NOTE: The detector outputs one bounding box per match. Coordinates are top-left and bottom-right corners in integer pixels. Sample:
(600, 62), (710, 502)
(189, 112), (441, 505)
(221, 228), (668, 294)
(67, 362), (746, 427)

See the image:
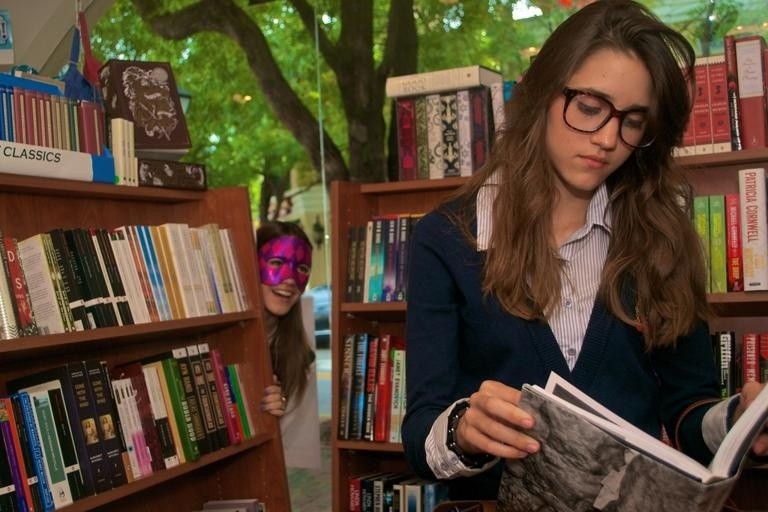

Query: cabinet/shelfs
(330, 149), (768, 511)
(0, 174), (294, 512)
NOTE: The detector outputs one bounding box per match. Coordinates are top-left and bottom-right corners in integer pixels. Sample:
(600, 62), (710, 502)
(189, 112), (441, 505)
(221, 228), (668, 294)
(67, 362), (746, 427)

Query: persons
(395, 1), (768, 511)
(259, 219), (317, 418)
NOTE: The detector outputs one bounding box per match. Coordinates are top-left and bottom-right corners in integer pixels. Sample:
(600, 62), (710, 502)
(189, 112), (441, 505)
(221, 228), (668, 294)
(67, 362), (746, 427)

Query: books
(140, 159), (208, 190)
(671, 33), (768, 158)
(1, 84), (104, 155)
(0, 342), (256, 512)
(691, 165), (768, 294)
(336, 329), (407, 444)
(105, 117), (139, 187)
(386, 63), (524, 181)
(348, 463), (448, 512)
(708, 326), (768, 400)
(495, 369), (768, 511)
(0, 220), (252, 341)
(344, 212), (427, 302)
(98, 57), (192, 163)
(201, 498), (266, 512)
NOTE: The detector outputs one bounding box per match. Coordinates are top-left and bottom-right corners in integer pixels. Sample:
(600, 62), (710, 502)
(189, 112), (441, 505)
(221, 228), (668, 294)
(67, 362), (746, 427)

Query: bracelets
(447, 403), (496, 470)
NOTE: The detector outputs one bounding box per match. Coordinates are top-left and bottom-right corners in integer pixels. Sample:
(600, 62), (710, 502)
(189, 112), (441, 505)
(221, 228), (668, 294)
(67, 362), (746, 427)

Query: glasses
(561, 86), (660, 149)
